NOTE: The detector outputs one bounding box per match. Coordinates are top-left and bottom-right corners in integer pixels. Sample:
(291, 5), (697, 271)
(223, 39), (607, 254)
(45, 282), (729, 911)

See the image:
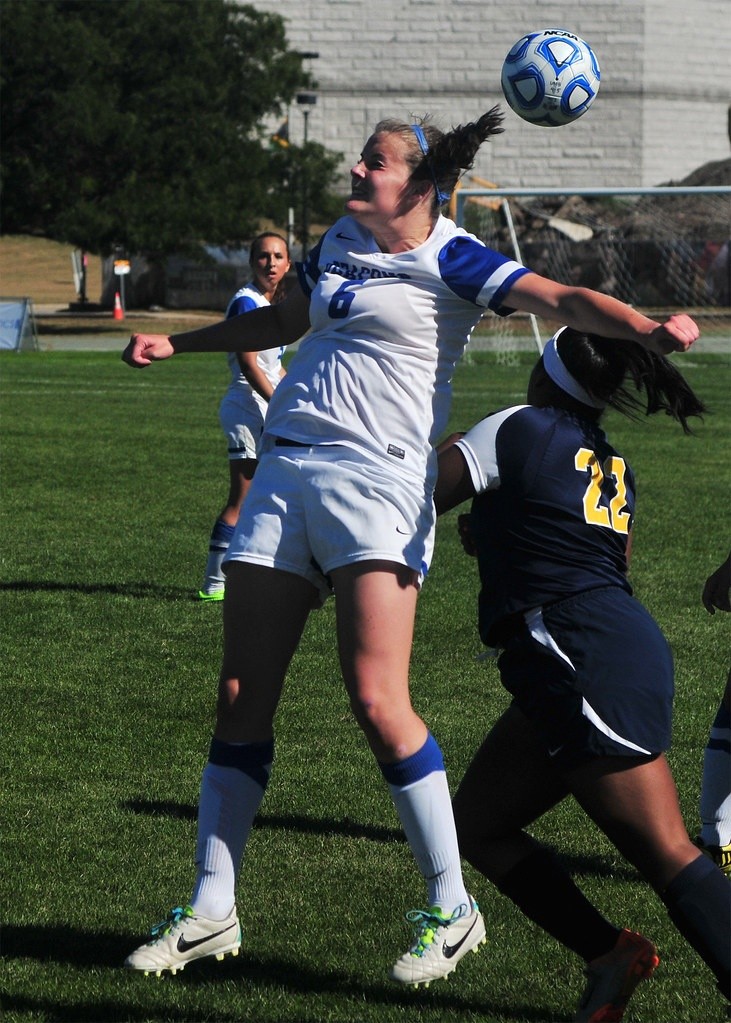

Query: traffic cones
(112, 292), (125, 319)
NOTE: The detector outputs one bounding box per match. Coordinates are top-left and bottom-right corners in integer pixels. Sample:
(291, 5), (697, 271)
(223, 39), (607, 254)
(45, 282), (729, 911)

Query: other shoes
(198, 580), (225, 601)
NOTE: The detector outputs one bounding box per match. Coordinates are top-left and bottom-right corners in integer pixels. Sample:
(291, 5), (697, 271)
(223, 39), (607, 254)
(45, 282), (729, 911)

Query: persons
(434, 324), (731, 1023)
(689, 551), (731, 871)
(198, 232), (287, 602)
(121, 104), (701, 989)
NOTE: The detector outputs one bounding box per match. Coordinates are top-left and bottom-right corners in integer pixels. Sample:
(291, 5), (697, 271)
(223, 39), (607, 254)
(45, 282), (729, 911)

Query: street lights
(297, 54), (318, 261)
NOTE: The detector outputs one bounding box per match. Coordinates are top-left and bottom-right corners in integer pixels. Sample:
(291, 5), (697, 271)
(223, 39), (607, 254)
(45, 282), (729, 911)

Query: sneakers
(573, 928), (659, 1023)
(124, 903), (242, 979)
(391, 894), (486, 991)
(691, 835), (731, 880)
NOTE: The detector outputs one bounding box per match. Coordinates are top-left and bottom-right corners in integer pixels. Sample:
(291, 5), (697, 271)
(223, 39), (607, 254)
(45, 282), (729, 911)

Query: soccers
(499, 29), (604, 129)
